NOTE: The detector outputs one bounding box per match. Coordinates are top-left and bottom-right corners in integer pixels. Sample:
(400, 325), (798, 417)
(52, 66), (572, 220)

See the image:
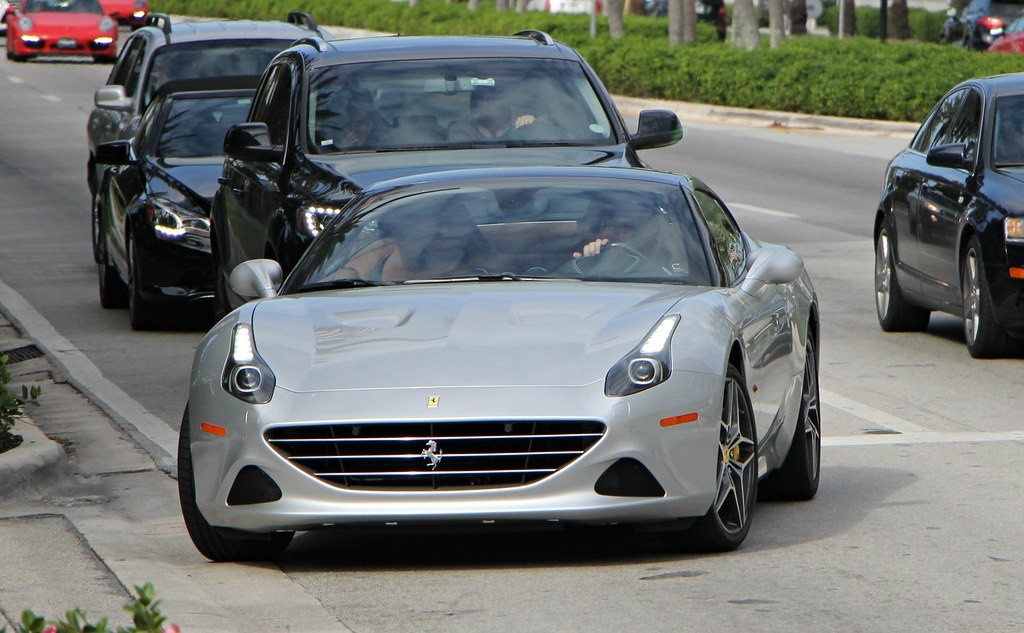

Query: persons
(553, 195), (671, 276)
(448, 87), (540, 144)
(337, 107), (383, 148)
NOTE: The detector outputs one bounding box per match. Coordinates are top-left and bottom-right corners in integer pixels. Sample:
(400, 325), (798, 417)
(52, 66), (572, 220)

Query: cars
(177, 165), (822, 562)
(873, 72), (1024, 359)
(0, 0), (148, 63)
(941, 0), (1024, 53)
(642, 0), (727, 41)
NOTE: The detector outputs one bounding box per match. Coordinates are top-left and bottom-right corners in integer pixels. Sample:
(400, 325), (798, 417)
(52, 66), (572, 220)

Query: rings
(524, 119), (529, 123)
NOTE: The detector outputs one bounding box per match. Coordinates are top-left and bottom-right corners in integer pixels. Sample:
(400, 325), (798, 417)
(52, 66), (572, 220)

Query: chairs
(383, 201), (503, 275)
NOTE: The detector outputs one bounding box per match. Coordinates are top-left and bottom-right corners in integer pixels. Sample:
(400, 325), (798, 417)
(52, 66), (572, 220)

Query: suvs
(87, 13), (683, 330)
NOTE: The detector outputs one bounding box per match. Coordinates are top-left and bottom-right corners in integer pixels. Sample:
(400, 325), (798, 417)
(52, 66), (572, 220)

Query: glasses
(575, 217), (614, 235)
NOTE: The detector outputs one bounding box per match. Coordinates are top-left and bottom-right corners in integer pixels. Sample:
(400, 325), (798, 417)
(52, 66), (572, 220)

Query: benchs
(372, 87), (466, 125)
(481, 222), (581, 270)
